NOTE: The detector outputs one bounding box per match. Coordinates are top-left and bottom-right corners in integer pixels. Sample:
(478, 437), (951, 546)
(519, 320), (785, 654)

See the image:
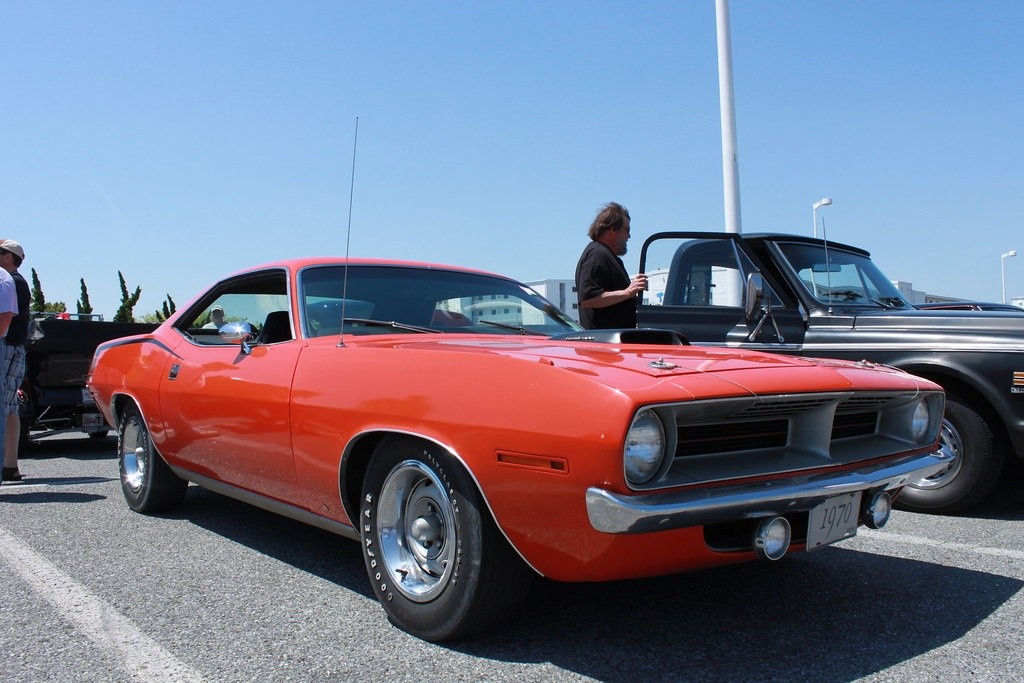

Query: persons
(202, 305), (228, 329)
(574, 202), (648, 330)
(0, 238), (31, 482)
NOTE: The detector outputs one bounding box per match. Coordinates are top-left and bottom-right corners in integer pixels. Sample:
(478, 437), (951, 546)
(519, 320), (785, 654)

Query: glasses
(621, 226), (630, 234)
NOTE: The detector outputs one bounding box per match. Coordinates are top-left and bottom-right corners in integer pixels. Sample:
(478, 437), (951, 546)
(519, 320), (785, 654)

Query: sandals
(1, 466), (22, 481)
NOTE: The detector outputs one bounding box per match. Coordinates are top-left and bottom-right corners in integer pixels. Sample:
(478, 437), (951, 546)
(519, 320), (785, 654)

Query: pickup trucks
(515, 226), (1024, 516)
(17, 310), (222, 459)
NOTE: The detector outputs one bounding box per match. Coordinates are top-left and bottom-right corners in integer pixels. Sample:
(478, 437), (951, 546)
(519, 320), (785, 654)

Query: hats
(0, 239), (25, 260)
(211, 305), (224, 313)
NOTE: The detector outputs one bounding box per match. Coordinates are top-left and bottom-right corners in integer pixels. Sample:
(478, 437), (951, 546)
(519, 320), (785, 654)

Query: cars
(83, 256), (959, 647)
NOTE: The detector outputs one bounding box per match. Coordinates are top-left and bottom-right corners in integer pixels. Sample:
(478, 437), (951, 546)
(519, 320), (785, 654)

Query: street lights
(1001, 251), (1017, 304)
(813, 197), (833, 238)
